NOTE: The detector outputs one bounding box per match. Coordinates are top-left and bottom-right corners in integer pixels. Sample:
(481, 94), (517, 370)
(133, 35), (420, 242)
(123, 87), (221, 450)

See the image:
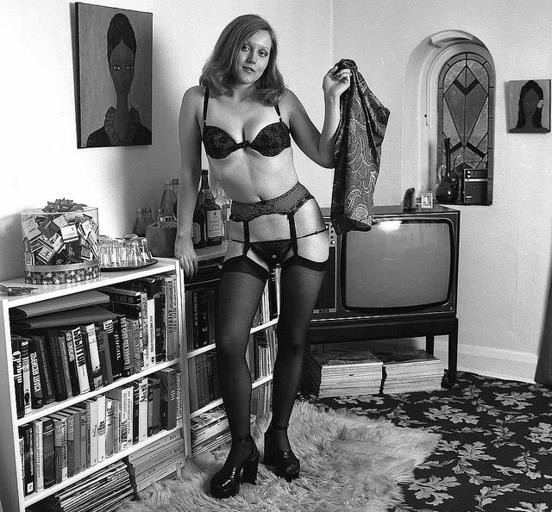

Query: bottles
(172, 176), (180, 202)
(200, 187), (225, 247)
(194, 169), (217, 209)
(189, 208), (209, 250)
(436, 138), (459, 203)
(161, 178), (178, 224)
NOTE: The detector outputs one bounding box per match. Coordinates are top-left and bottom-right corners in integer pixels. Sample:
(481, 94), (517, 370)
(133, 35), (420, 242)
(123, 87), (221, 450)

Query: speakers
(313, 206), (338, 320)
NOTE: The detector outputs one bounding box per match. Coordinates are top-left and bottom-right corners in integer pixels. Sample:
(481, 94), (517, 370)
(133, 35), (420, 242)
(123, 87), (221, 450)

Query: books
(189, 352), (229, 405)
(304, 343), (446, 399)
(6, 276), (194, 420)
(2, 363), (180, 507)
(245, 332), (297, 379)
(192, 385), (282, 456)
(183, 277), (217, 335)
(250, 278), (269, 322)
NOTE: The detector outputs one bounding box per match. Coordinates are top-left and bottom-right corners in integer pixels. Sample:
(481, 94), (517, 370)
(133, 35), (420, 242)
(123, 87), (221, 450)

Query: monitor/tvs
(339, 204), (461, 318)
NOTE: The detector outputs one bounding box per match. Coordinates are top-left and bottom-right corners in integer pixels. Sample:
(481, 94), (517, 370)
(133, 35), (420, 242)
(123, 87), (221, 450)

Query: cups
(99, 233), (152, 268)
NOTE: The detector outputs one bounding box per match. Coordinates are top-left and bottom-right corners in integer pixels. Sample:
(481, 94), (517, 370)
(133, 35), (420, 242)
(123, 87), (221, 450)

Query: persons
(505, 82), (550, 133)
(77, 2), (151, 141)
(174, 14), (353, 497)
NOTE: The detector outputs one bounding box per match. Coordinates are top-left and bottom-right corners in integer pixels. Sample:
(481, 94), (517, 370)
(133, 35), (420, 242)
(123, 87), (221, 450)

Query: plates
(100, 259), (158, 272)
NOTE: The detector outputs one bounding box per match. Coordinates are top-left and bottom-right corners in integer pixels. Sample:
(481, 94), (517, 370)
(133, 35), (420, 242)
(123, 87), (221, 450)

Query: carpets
(122, 401), (441, 511)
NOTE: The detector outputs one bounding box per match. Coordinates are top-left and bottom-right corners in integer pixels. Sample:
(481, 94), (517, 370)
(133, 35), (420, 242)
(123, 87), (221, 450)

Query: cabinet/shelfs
(156, 240), (281, 457)
(304, 318), (458, 398)
(1, 255), (188, 509)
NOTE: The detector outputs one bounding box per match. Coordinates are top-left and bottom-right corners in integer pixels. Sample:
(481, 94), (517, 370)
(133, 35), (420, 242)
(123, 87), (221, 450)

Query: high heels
(263, 426), (300, 481)
(210, 432), (260, 499)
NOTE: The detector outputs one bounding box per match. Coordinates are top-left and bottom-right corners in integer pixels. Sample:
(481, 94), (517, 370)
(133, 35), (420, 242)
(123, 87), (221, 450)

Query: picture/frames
(420, 193), (433, 208)
